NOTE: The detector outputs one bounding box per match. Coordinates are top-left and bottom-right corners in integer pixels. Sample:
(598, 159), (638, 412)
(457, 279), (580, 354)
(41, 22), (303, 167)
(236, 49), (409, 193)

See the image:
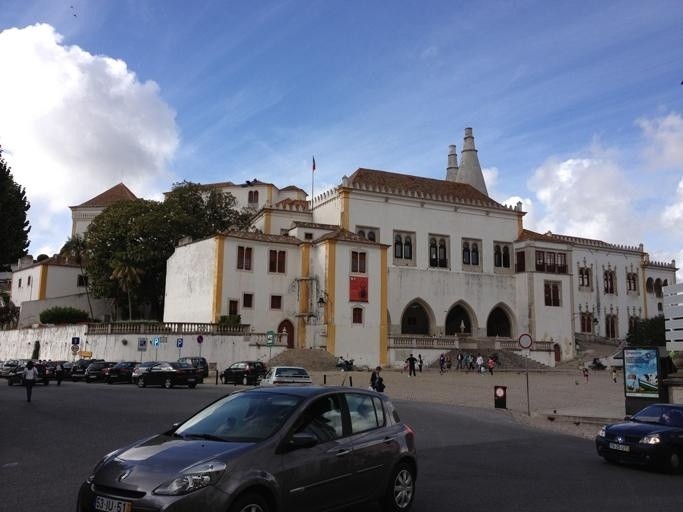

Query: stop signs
(518, 334), (532, 348)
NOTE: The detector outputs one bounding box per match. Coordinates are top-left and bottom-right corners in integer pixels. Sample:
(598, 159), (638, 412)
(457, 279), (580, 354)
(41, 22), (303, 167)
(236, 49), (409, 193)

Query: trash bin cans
(495, 386), (507, 409)
(208, 363), (216, 376)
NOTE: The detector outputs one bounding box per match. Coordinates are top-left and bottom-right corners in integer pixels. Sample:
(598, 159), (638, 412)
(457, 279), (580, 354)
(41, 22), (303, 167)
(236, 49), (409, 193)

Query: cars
(75, 385), (418, 511)
(258, 366), (312, 387)
(595, 403), (682, 474)
(0, 357), (208, 389)
(220, 361), (267, 386)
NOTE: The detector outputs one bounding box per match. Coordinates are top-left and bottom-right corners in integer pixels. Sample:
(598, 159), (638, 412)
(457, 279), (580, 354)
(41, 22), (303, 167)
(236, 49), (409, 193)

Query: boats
(638, 378), (658, 391)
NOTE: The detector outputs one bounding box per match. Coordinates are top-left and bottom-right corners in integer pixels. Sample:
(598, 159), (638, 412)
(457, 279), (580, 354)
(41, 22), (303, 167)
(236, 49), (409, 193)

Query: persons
(39, 359), (63, 385)
(404, 361), (408, 372)
(24, 361), (38, 402)
(439, 351), (495, 375)
(338, 356), (347, 371)
(613, 370), (617, 383)
(583, 368), (589, 383)
(417, 354), (423, 372)
(371, 367), (381, 391)
(376, 377), (385, 392)
(407, 354), (417, 377)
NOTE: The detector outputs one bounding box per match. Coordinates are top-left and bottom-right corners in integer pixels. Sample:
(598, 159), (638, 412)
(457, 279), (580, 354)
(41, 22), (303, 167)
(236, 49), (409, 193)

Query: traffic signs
(267, 330), (273, 347)
(77, 351), (92, 357)
(154, 338), (160, 347)
(177, 338), (183, 348)
